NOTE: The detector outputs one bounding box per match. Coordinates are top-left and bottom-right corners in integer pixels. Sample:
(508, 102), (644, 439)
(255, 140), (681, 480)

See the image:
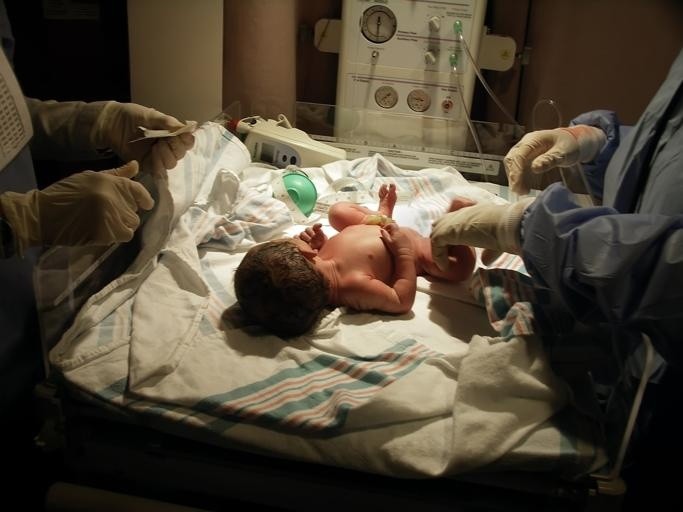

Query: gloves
(430, 196), (538, 273)
(503, 124), (607, 196)
(1, 159), (155, 258)
(25, 96), (195, 169)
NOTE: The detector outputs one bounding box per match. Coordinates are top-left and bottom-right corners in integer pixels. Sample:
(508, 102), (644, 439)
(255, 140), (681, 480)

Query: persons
(234, 184), (477, 338)
(429, 47), (683, 512)
(0, 1), (196, 512)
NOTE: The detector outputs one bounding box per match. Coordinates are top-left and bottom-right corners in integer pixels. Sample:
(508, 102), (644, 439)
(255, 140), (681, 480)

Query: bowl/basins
(280, 173), (317, 217)
